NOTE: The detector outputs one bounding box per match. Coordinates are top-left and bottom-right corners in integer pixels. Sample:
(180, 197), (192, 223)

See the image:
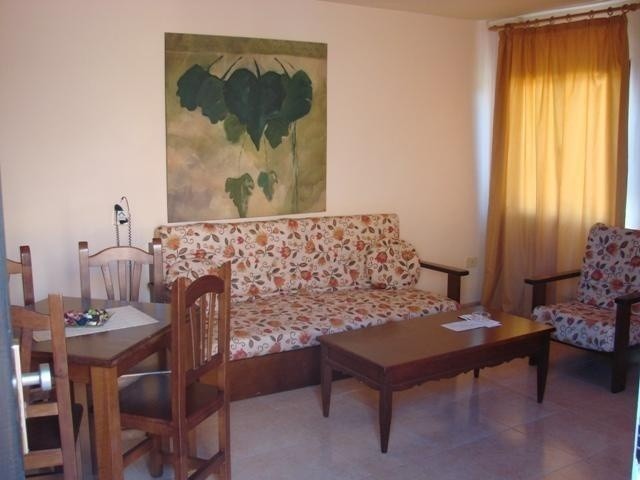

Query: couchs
(151, 214), (468, 402)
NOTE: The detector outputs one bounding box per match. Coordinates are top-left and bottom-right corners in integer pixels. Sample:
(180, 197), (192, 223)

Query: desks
(8, 291), (204, 476)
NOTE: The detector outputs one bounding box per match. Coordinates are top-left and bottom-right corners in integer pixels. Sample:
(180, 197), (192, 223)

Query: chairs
(5, 241), (38, 313)
(72, 238), (168, 304)
(8, 292), (89, 480)
(106, 259), (233, 475)
(525, 223), (640, 393)
